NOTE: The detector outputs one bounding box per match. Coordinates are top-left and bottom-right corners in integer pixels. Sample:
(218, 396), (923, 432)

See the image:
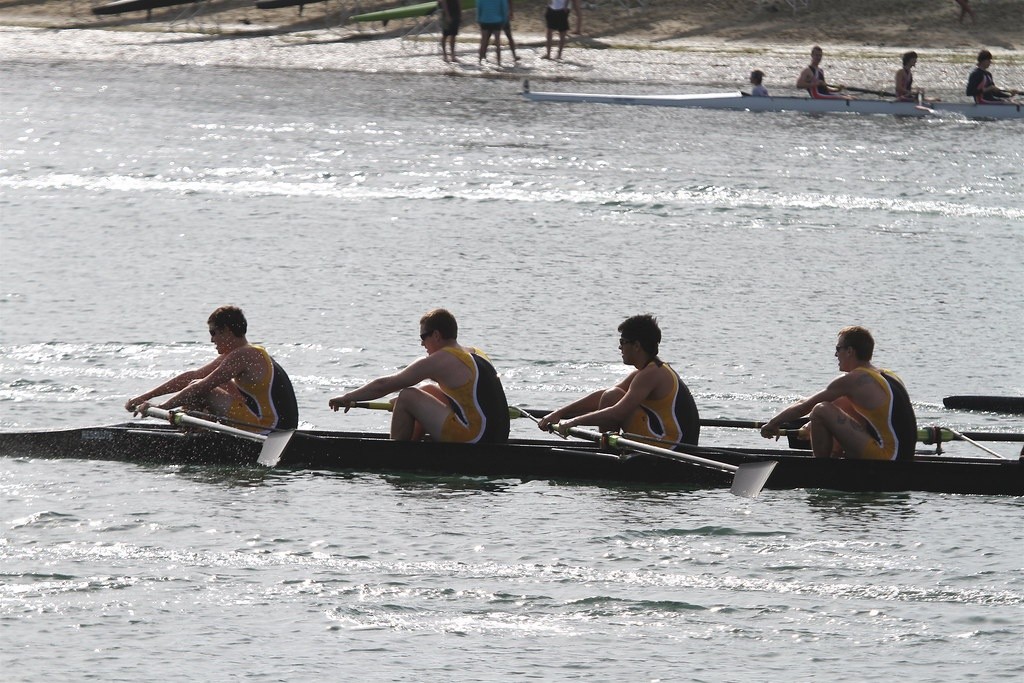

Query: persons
(966, 50), (1014, 104)
(896, 51), (924, 101)
(760, 326), (917, 460)
(749, 70), (768, 95)
(126, 306), (298, 434)
(955, 0), (976, 27)
(328, 308), (510, 442)
(797, 45), (859, 98)
(438, 0), (582, 66)
(537, 315), (700, 452)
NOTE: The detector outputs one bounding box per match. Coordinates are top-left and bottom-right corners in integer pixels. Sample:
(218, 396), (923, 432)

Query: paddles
(538, 421), (780, 499)
(825, 83), (896, 97)
(998, 88), (1024, 96)
(128, 403), (298, 469)
(335, 401), (813, 449)
(762, 426), (1024, 446)
(916, 92), (930, 111)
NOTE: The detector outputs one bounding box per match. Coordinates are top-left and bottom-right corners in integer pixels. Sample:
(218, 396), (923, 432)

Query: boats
(942, 394), (1024, 415)
(90, 0), (330, 16)
(349, 0), (477, 22)
(518, 78), (1024, 124)
(0, 418), (1024, 500)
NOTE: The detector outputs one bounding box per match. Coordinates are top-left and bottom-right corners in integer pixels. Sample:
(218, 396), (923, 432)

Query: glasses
(619, 338), (631, 345)
(835, 345), (845, 352)
(209, 328), (219, 337)
(420, 333), (428, 340)
